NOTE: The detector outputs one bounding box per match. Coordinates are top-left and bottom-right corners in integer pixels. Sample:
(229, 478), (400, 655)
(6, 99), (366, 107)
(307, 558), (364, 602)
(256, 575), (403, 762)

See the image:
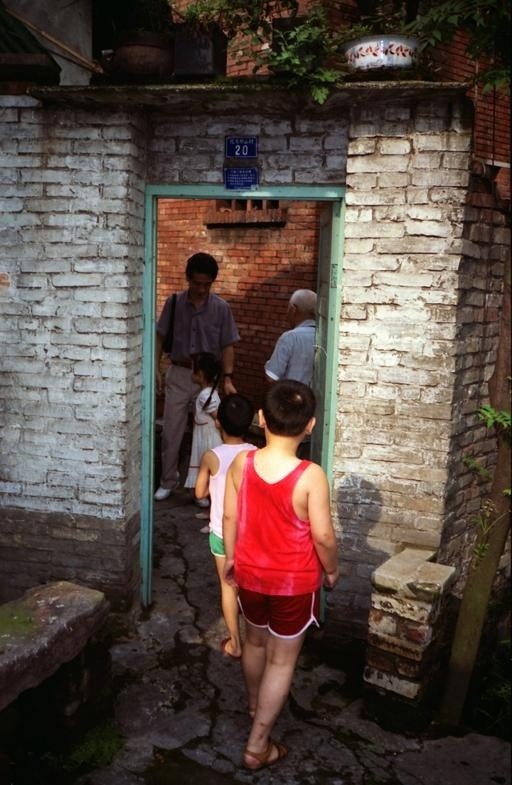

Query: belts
(172, 359), (192, 369)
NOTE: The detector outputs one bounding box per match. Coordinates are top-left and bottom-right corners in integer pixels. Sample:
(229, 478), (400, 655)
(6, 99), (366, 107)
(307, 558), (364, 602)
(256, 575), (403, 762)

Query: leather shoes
(200, 526), (208, 533)
(195, 512), (209, 519)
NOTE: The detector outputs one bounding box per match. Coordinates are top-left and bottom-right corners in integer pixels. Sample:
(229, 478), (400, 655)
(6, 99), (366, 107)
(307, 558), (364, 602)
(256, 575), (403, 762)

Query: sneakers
(154, 479), (179, 503)
(181, 488), (210, 508)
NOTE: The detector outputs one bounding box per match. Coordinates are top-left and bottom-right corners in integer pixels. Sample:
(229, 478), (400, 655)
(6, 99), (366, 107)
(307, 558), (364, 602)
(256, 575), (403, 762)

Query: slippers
(221, 637), (242, 659)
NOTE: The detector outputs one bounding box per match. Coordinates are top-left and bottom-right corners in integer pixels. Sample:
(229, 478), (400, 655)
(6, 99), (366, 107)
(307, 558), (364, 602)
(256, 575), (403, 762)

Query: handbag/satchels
(162, 293), (177, 355)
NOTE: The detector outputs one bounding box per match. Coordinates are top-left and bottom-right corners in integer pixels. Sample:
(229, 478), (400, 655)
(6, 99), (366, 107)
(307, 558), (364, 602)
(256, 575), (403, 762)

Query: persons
(184, 353), (222, 535)
(263, 289), (320, 463)
(194, 392), (259, 659)
(221, 377), (342, 772)
(154, 251), (241, 502)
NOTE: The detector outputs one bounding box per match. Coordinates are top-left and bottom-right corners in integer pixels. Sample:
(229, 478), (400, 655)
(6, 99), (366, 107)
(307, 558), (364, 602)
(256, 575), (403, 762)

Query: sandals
(245, 701), (288, 773)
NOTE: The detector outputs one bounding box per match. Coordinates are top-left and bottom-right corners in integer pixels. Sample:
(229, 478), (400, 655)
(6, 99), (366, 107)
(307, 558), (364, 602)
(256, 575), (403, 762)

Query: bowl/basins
(336, 32), (423, 78)
(112, 43), (175, 82)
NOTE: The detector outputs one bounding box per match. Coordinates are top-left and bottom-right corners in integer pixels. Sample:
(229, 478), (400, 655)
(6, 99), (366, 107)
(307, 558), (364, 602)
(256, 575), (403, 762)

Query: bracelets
(223, 372), (234, 379)
